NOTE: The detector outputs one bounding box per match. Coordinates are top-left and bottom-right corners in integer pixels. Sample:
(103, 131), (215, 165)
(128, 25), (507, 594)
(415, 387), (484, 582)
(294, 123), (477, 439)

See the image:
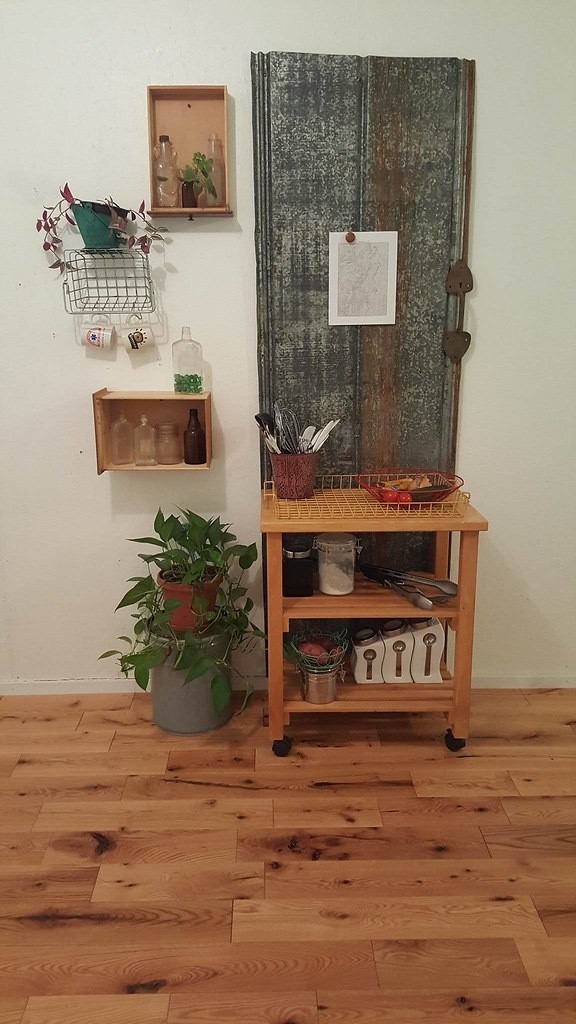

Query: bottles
(183, 409), (207, 465)
(111, 409), (134, 465)
(155, 423), (182, 464)
(204, 130), (225, 207)
(153, 135), (179, 208)
(133, 414), (157, 466)
(172, 326), (203, 395)
(349, 617), (447, 684)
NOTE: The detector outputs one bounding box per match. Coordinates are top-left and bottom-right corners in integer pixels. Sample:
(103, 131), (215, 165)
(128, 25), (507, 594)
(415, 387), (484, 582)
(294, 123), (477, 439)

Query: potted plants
(96, 504), (269, 717)
(36, 183), (170, 278)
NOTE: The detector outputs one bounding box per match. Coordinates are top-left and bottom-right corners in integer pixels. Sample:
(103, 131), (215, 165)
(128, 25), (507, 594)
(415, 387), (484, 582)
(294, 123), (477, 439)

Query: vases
(270, 453), (318, 499)
(181, 152), (217, 209)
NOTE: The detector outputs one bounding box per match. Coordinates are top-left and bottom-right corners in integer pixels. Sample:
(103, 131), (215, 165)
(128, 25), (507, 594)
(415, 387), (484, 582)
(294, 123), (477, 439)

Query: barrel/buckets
(146, 607), (234, 735)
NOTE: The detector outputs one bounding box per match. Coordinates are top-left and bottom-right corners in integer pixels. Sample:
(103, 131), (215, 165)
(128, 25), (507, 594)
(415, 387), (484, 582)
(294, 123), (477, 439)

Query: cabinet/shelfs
(92, 387), (213, 476)
(259, 489), (488, 758)
(146, 84), (234, 221)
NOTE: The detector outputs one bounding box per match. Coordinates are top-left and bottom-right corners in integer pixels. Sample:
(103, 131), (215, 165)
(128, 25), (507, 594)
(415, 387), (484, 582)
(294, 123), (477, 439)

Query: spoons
(397, 580), (450, 601)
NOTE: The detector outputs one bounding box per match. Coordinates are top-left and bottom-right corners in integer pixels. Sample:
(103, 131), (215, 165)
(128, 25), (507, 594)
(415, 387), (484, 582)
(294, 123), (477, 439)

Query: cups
(119, 314), (154, 353)
(313, 532), (362, 595)
(295, 663), (347, 704)
(80, 314), (115, 352)
(283, 543), (314, 597)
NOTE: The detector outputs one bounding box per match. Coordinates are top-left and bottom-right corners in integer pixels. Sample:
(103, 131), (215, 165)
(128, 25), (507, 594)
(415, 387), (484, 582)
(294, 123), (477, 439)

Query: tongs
(362, 563), (459, 610)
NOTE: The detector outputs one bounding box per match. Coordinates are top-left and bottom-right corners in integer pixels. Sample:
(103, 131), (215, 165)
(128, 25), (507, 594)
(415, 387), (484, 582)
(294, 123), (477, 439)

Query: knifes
(301, 418), (341, 453)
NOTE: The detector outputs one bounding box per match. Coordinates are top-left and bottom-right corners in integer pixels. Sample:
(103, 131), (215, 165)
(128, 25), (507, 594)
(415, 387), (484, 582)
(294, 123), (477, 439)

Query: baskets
(263, 473), (471, 519)
(291, 628), (350, 668)
(62, 247), (156, 321)
(356, 467), (464, 509)
